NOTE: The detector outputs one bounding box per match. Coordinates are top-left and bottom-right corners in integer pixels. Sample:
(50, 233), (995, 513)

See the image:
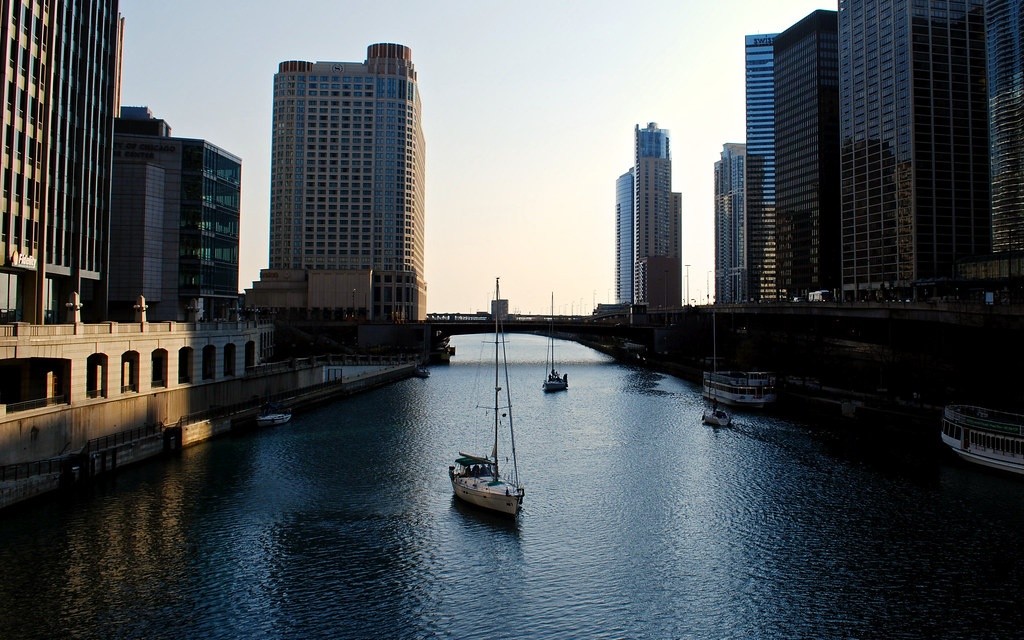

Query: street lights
(685, 264), (692, 306)
(351, 289), (360, 320)
(665, 269), (669, 322)
(707, 270), (712, 305)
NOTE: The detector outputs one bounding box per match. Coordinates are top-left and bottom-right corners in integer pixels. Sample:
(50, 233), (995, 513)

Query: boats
(702, 368), (778, 408)
(257, 404), (292, 425)
(415, 364), (431, 378)
(939, 405), (1024, 476)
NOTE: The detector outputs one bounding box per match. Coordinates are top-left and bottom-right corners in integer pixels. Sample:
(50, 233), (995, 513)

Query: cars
(791, 296), (806, 304)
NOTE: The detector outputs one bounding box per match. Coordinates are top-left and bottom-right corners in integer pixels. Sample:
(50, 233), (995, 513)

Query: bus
(809, 290), (831, 303)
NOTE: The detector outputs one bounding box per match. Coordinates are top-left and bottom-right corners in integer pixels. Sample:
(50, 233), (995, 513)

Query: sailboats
(698, 297), (730, 427)
(543, 293), (568, 391)
(449, 275), (526, 519)
